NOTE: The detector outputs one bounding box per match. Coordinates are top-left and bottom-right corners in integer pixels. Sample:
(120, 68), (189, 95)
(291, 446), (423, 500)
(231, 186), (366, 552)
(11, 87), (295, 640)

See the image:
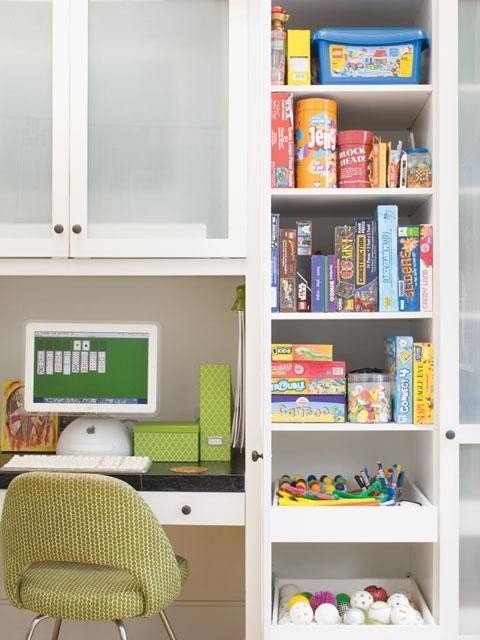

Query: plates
(0, 0), (271, 276)
(246, 0), (480, 640)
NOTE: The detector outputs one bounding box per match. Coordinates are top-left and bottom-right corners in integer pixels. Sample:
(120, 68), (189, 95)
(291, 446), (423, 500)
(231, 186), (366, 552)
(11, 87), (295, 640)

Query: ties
(23, 321), (166, 455)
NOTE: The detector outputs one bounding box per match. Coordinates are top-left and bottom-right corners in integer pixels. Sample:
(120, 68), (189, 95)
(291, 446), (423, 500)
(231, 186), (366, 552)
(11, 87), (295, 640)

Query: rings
(354, 458), (404, 500)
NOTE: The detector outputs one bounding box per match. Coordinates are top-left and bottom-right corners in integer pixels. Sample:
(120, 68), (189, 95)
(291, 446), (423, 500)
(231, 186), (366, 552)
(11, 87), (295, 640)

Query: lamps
(0, 473), (189, 640)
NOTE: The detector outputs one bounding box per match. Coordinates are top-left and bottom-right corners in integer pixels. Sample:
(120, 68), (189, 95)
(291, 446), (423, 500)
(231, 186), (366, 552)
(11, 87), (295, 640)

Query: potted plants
(1, 378), (61, 453)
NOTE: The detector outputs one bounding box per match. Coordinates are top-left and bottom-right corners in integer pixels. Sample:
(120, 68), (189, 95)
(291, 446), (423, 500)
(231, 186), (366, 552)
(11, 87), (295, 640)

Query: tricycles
(73, 340), (91, 351)
(37, 351), (106, 374)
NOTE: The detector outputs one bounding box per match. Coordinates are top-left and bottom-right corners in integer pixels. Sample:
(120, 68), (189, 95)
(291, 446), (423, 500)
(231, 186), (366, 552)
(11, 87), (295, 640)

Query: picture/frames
(1, 441), (248, 530)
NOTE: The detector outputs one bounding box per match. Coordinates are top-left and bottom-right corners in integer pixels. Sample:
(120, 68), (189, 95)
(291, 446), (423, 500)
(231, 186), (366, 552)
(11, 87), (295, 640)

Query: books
(310, 24), (432, 86)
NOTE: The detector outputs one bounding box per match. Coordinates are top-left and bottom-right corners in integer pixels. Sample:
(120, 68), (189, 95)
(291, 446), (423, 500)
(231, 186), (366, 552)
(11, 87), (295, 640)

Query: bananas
(1, 453), (153, 475)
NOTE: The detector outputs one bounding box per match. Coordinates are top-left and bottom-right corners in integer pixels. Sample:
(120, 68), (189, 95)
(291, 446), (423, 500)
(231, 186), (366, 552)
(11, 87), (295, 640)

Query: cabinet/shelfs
(295, 96), (338, 189)
(336, 128), (377, 189)
(347, 373), (393, 423)
(406, 147), (432, 189)
